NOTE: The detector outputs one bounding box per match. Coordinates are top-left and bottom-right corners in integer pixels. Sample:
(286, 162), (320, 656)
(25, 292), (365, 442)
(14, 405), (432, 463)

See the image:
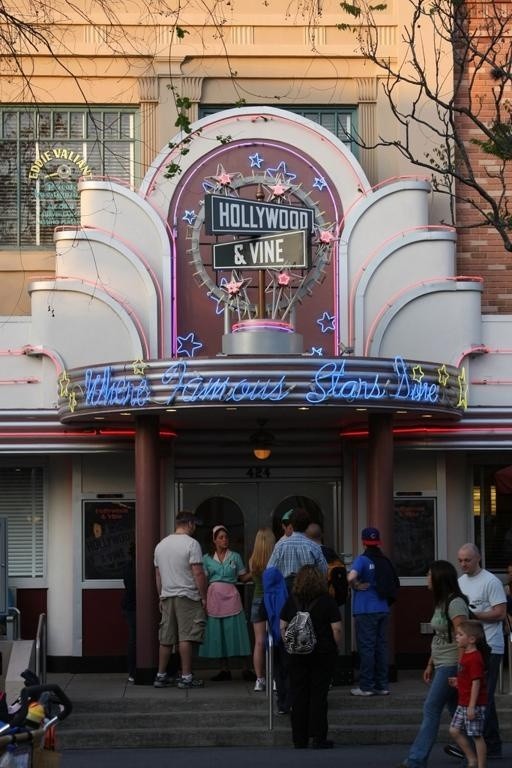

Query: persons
(400, 543), (507, 768)
(350, 527), (390, 695)
(277, 564), (345, 748)
(152, 510), (293, 691)
(306, 522), (348, 606)
(266, 508), (328, 714)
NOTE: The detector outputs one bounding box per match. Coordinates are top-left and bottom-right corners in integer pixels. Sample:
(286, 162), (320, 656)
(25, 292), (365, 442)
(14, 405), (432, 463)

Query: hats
(360, 526), (384, 547)
(211, 524), (229, 543)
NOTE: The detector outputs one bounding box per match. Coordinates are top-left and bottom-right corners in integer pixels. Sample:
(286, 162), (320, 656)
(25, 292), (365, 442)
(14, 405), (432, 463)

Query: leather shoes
(312, 739), (335, 750)
(209, 669), (233, 682)
(240, 669), (257, 682)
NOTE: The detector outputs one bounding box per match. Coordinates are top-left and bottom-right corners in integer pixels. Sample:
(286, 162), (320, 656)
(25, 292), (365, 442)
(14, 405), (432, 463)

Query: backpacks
(444, 591), (492, 672)
(362, 550), (402, 602)
(282, 593), (322, 656)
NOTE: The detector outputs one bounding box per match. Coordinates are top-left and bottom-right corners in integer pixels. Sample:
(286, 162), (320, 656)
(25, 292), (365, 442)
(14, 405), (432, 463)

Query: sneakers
(272, 678), (277, 691)
(177, 672), (205, 690)
(370, 684), (390, 696)
(486, 746), (504, 760)
(443, 741), (466, 759)
(153, 672), (182, 689)
(350, 687), (374, 697)
(253, 677), (266, 692)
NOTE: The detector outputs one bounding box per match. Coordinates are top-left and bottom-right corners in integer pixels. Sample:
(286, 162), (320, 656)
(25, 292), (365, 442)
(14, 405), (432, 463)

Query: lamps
(251, 425), (275, 461)
